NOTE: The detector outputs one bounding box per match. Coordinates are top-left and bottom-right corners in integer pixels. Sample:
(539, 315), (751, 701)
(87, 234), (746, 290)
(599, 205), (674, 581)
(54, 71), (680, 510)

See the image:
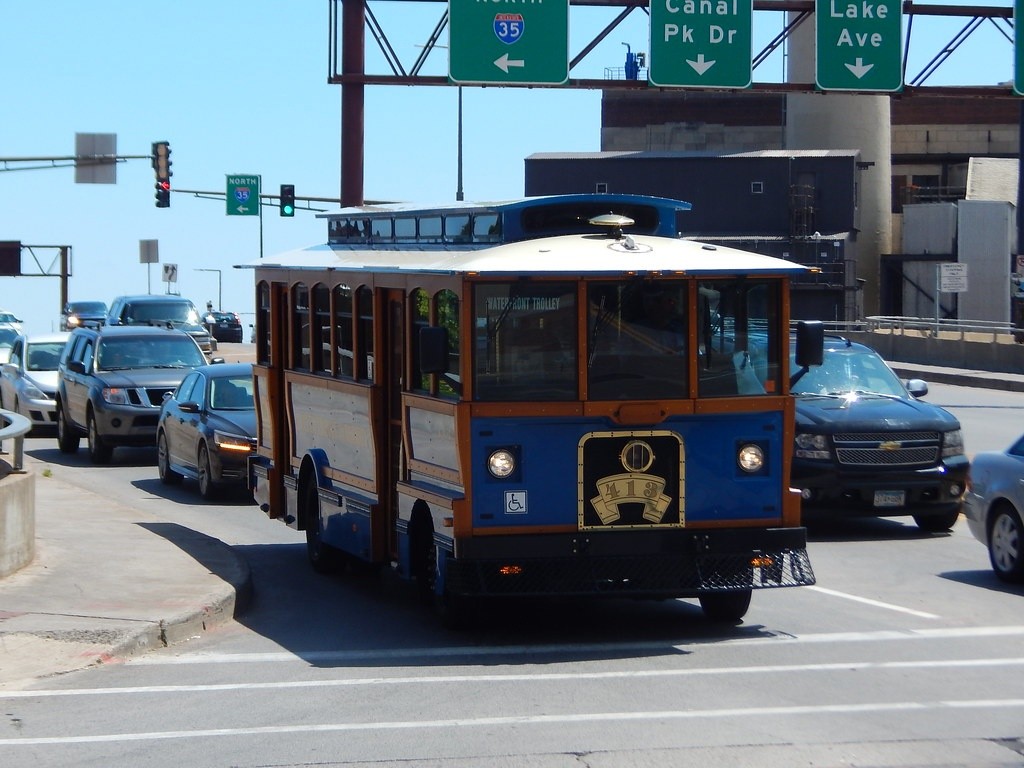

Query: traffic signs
(226, 174), (259, 216)
(448, 1), (569, 85)
(648, 1), (752, 90)
(815, 1), (903, 92)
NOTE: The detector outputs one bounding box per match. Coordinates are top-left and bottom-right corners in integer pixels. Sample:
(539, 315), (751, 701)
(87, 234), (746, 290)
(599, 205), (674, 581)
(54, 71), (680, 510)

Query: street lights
(195, 268), (222, 311)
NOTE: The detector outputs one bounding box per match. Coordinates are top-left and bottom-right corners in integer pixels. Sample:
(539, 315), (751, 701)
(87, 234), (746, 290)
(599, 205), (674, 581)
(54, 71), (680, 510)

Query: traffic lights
(280, 184), (295, 217)
(154, 141), (170, 207)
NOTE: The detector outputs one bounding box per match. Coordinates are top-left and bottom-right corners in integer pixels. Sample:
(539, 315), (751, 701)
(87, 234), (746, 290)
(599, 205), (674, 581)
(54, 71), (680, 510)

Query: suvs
(103, 294), (213, 357)
(713, 329), (970, 532)
(55, 319), (225, 465)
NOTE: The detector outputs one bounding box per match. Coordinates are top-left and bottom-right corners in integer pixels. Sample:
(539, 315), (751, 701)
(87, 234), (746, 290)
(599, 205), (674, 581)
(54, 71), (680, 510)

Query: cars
(249, 323), (256, 343)
(0, 311), (24, 364)
(156, 360), (257, 499)
(202, 311), (242, 343)
(0, 332), (73, 437)
(964, 432), (1024, 586)
(59, 301), (109, 328)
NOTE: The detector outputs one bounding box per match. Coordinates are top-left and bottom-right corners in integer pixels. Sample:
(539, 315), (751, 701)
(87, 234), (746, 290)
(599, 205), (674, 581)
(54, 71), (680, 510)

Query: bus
(232, 194), (816, 621)
